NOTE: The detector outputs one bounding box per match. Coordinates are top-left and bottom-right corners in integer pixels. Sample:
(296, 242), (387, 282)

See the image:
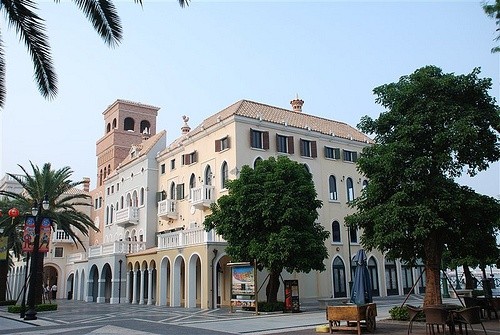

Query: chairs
(450, 306), (487, 335)
(425, 306), (454, 335)
(405, 304), (427, 335)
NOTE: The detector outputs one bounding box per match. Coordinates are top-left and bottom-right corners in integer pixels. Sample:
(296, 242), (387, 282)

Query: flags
(0, 235), (9, 261)
(39, 217), (51, 253)
(22, 217), (35, 252)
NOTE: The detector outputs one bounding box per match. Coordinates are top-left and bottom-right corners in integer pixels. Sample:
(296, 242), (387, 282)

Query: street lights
(22, 190), (49, 319)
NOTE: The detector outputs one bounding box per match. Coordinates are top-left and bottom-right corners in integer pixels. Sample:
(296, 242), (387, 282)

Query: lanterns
(8, 208), (20, 220)
(0, 211), (3, 218)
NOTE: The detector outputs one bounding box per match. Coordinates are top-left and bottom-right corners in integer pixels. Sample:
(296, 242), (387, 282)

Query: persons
(42, 282), (45, 288)
(43, 284), (49, 300)
(50, 284), (57, 300)
